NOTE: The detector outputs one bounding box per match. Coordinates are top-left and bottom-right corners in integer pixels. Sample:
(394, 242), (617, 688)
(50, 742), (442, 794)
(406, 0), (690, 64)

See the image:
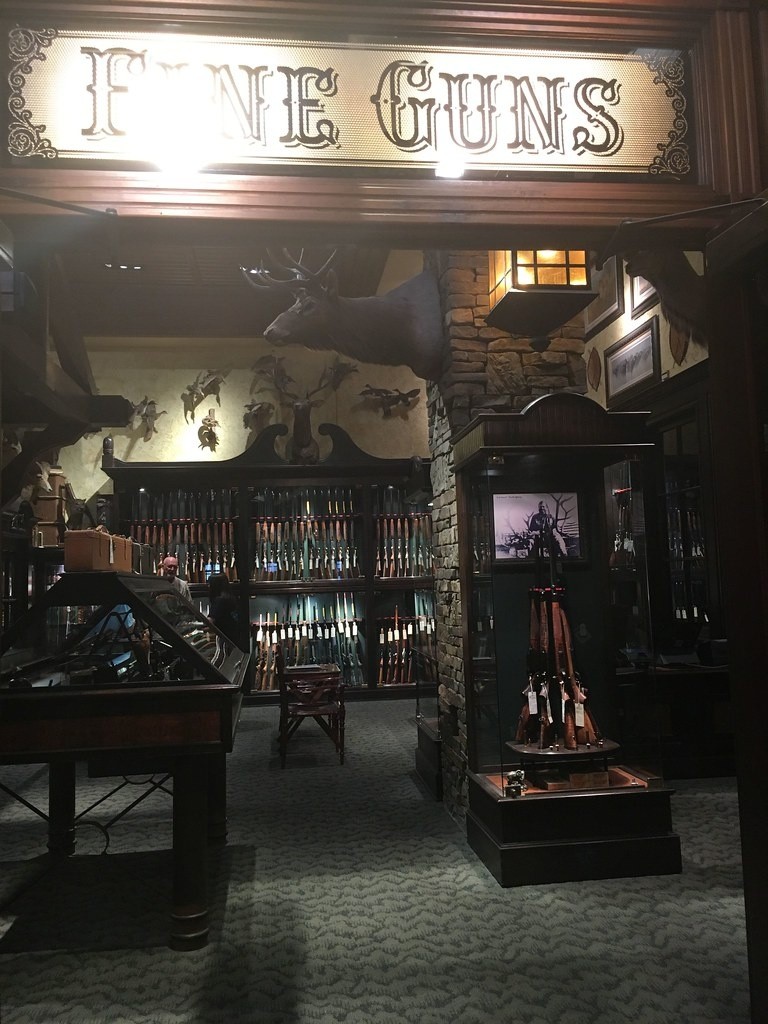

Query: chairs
(276, 641), (347, 769)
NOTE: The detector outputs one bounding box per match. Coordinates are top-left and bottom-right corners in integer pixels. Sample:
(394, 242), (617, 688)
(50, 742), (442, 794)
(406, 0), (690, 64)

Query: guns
(413, 509), (437, 680)
(126, 485), (411, 692)
(471, 507), (494, 658)
(514, 588), (604, 750)
(601, 461), (711, 640)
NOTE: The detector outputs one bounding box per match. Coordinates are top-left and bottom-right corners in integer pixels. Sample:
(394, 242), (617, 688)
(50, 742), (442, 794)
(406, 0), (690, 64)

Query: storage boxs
(63, 530), (132, 572)
(133, 543), (158, 575)
(29, 547), (64, 565)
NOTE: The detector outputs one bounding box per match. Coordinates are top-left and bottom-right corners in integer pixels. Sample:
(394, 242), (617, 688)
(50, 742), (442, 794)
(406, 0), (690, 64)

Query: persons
(65, 624), (88, 649)
(190, 572), (240, 656)
(527, 500), (555, 549)
(152, 556), (193, 606)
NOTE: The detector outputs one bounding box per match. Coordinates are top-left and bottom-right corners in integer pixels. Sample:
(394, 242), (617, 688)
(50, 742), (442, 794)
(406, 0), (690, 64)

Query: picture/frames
(584, 255), (625, 343)
(629, 275), (660, 320)
(603, 313), (662, 409)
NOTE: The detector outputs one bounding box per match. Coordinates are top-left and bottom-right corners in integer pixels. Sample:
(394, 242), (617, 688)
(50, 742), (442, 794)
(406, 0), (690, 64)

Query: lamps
(483, 250), (600, 354)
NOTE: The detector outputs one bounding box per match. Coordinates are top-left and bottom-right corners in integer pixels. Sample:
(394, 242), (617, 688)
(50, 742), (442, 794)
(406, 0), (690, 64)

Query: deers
(237, 245), (446, 384)
